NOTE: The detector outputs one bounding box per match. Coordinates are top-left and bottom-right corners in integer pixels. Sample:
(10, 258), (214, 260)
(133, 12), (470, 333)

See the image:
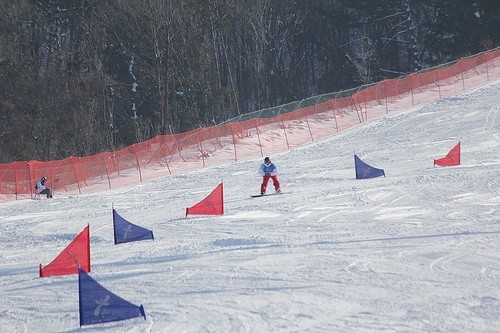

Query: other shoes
(277, 189), (282, 193)
(261, 191), (266, 195)
(46, 194), (53, 198)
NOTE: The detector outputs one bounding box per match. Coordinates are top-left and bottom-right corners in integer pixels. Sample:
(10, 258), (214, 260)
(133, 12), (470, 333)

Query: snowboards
(250, 191), (289, 198)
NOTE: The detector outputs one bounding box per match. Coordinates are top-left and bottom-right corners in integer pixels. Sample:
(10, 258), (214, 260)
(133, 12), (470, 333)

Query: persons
(34, 176), (53, 199)
(258, 157), (282, 196)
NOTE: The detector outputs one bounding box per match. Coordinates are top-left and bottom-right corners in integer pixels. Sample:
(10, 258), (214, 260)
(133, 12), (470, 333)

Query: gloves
(266, 172), (271, 176)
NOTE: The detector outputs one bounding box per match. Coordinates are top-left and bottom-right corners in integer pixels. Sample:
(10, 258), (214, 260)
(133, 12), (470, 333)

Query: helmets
(265, 157), (269, 162)
(42, 176), (47, 181)
